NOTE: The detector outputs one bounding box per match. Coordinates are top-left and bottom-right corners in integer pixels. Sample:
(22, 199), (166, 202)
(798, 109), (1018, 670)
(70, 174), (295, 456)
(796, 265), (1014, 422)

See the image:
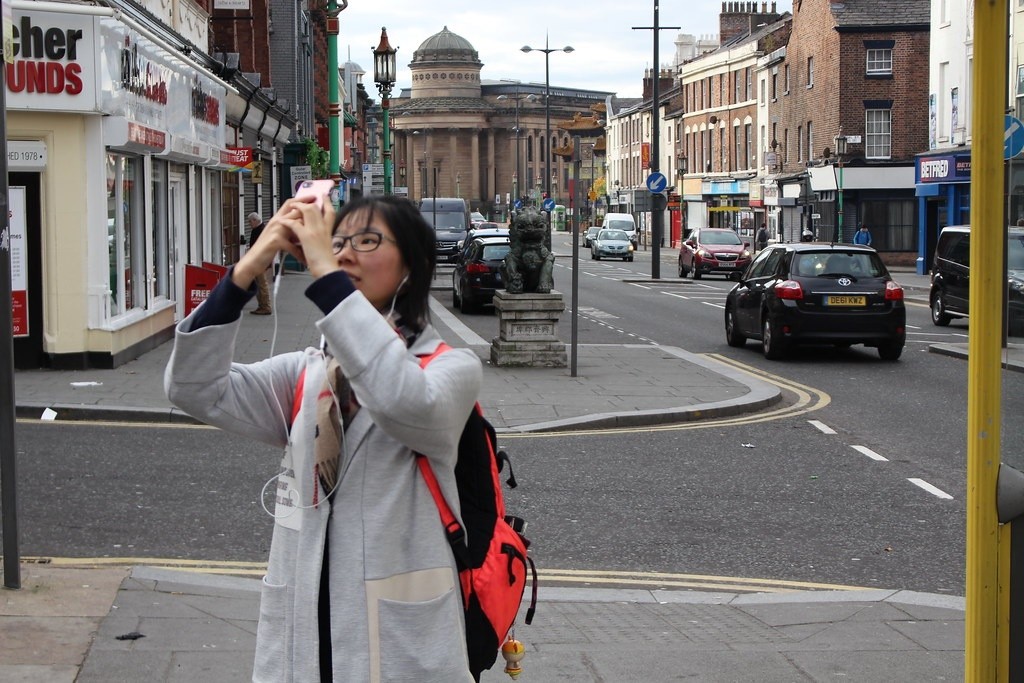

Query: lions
(497, 205), (556, 294)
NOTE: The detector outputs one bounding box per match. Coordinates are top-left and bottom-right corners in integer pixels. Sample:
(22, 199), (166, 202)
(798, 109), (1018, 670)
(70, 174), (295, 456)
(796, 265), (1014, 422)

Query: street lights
(833, 126), (848, 242)
(536, 174), (544, 201)
(520, 27), (575, 250)
(370, 26), (400, 196)
(512, 171), (518, 203)
(496, 80), (536, 215)
(456, 170), (462, 197)
(552, 172), (559, 204)
(677, 148), (687, 246)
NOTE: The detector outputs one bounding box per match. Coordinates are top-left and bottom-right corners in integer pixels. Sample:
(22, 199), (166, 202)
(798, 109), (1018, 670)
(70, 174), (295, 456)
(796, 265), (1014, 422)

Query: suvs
(677, 226), (752, 279)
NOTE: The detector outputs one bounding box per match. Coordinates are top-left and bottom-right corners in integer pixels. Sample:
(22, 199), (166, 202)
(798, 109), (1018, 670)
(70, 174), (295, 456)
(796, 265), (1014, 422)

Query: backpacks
(292, 343), (537, 683)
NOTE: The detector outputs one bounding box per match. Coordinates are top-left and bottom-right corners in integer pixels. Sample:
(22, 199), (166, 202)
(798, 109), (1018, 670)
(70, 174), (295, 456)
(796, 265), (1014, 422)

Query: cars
(447, 236), (512, 314)
(582, 226), (603, 248)
(590, 228), (634, 263)
(724, 242), (907, 360)
(451, 229), (510, 278)
(469, 213), (489, 230)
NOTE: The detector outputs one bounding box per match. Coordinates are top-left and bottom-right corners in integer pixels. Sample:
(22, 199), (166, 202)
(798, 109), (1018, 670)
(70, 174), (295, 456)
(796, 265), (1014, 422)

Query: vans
(929, 225), (1024, 337)
(602, 213), (641, 251)
(414, 197), (473, 266)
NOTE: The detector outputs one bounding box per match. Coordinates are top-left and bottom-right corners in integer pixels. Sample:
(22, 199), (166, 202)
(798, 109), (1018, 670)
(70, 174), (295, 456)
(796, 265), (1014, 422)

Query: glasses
(331, 232), (399, 255)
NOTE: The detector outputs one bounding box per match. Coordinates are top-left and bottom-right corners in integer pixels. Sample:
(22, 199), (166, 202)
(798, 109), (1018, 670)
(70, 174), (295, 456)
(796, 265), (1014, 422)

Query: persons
(247, 212), (274, 315)
(755, 223), (771, 251)
(167, 197), (483, 683)
(853, 223), (872, 246)
(801, 227), (814, 242)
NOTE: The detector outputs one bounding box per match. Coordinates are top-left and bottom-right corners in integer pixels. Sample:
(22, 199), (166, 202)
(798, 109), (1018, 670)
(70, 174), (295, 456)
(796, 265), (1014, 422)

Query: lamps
(180, 45), (193, 55)
(413, 131), (420, 135)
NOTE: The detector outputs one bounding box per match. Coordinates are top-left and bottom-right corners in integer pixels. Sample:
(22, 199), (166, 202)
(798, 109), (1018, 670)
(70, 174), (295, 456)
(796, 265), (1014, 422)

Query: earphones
(402, 274), (410, 283)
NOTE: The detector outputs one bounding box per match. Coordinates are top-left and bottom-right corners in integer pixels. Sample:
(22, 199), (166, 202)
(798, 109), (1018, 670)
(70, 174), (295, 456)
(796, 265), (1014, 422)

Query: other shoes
(249, 309), (271, 315)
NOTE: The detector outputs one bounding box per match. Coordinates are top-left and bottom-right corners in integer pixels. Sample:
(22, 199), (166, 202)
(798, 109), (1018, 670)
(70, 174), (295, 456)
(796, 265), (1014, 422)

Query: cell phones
(288, 178), (335, 246)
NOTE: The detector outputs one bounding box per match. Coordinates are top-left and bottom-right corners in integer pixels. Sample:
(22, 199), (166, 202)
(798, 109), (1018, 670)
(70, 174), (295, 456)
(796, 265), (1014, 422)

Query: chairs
(800, 258), (817, 277)
(602, 233), (610, 240)
(822, 255), (849, 273)
(614, 233), (626, 239)
